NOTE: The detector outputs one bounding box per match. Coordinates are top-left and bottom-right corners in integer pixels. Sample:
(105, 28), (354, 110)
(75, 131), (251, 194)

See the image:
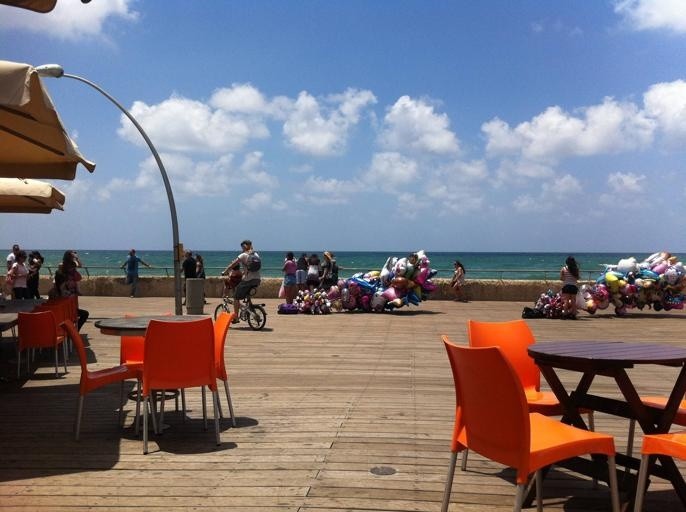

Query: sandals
(463, 297), (468, 302)
(454, 297), (460, 301)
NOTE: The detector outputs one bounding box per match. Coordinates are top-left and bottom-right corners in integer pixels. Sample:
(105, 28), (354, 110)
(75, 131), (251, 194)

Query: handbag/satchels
(5, 263), (19, 289)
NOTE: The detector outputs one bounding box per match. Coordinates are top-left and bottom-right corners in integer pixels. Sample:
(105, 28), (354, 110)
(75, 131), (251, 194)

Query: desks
(521, 340), (686, 511)
(94, 315), (206, 432)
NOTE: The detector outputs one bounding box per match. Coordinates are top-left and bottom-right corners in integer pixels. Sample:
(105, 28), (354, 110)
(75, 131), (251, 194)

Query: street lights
(30, 64), (183, 317)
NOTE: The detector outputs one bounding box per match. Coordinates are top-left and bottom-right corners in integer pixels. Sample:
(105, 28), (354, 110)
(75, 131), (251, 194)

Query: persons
(179, 249), (195, 306)
(306, 253), (321, 291)
(451, 260), (468, 302)
(54, 250), (82, 297)
(280, 252), (296, 304)
(48, 271), (88, 332)
(27, 250), (43, 299)
(224, 262), (242, 297)
(222, 240), (260, 323)
(192, 254), (206, 304)
(5, 245), (19, 271)
(559, 257), (580, 320)
(330, 259), (342, 286)
(317, 251), (332, 290)
(27, 256), (43, 278)
(119, 249), (151, 298)
(6, 250), (28, 300)
(296, 253), (306, 292)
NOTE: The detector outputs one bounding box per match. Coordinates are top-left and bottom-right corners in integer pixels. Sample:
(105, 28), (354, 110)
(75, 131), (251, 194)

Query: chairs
(634, 435), (686, 511)
(17, 293), (79, 378)
(120, 313), (186, 429)
(135, 316), (221, 455)
(440, 336), (620, 512)
(181, 310), (238, 432)
(57, 319), (143, 443)
(640, 396), (686, 427)
(461, 321), (600, 491)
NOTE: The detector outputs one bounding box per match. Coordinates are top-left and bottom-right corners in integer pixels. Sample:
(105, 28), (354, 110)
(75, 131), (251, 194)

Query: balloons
(292, 286), (338, 314)
(337, 249), (437, 312)
(522, 289), (563, 319)
(578, 252), (686, 315)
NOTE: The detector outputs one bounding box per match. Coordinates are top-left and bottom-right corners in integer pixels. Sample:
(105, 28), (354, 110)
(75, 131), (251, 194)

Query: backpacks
(246, 253), (261, 272)
(308, 266), (319, 282)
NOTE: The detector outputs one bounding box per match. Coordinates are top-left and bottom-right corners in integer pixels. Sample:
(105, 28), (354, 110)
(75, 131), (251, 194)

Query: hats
(324, 251), (332, 260)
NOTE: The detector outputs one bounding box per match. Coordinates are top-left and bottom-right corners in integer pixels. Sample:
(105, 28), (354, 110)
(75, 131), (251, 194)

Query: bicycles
(214, 271), (268, 331)
